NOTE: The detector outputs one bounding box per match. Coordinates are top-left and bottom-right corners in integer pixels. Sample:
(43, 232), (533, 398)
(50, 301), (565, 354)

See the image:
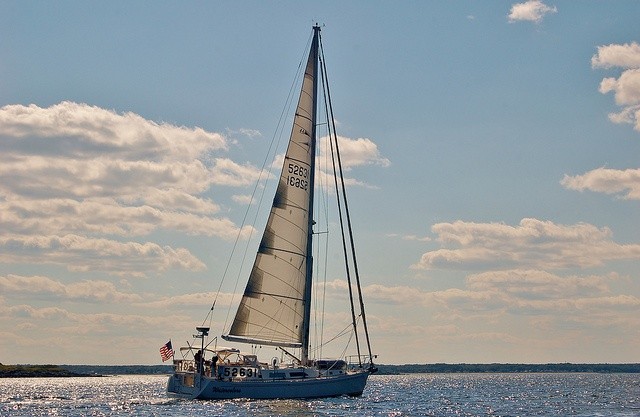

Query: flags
(159, 339), (173, 362)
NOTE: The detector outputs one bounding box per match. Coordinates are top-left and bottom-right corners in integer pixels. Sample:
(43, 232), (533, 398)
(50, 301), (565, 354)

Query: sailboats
(166, 19), (379, 400)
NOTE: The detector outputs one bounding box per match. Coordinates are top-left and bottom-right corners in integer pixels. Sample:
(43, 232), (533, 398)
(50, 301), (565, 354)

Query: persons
(194, 350), (207, 375)
